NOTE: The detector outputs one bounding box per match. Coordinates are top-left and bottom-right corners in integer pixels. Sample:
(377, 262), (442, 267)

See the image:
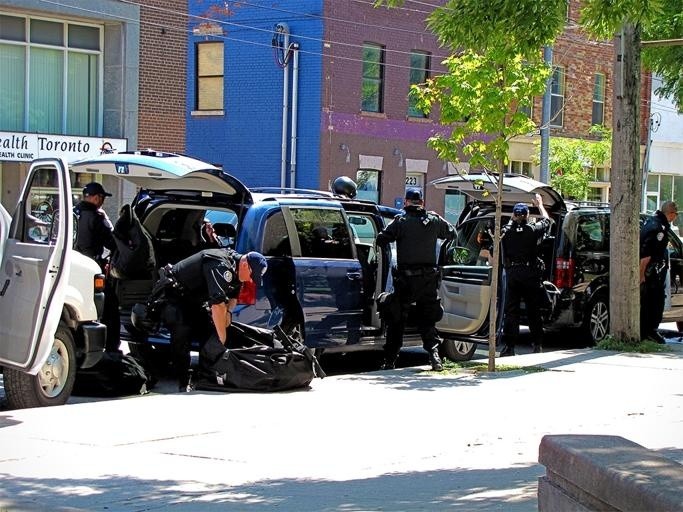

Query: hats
(514, 203), (528, 216)
(247, 251), (267, 286)
(405, 187), (423, 201)
(82, 182), (113, 197)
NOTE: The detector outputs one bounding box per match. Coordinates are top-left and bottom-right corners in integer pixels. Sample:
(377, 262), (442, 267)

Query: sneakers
(499, 346), (514, 356)
(531, 343), (542, 352)
(648, 329), (666, 345)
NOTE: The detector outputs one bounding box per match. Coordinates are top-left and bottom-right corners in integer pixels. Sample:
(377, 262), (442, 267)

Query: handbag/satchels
(107, 204), (170, 282)
(192, 319), (315, 393)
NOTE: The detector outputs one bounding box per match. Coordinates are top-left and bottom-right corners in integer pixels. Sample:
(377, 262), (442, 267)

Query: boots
(429, 348), (443, 371)
(378, 354), (397, 369)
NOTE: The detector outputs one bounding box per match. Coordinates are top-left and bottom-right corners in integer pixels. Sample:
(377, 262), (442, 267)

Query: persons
(147, 248), (268, 392)
(73, 181), (115, 257)
(374, 186), (457, 373)
(477, 191), (552, 355)
(311, 261), (361, 344)
(640, 200), (678, 344)
